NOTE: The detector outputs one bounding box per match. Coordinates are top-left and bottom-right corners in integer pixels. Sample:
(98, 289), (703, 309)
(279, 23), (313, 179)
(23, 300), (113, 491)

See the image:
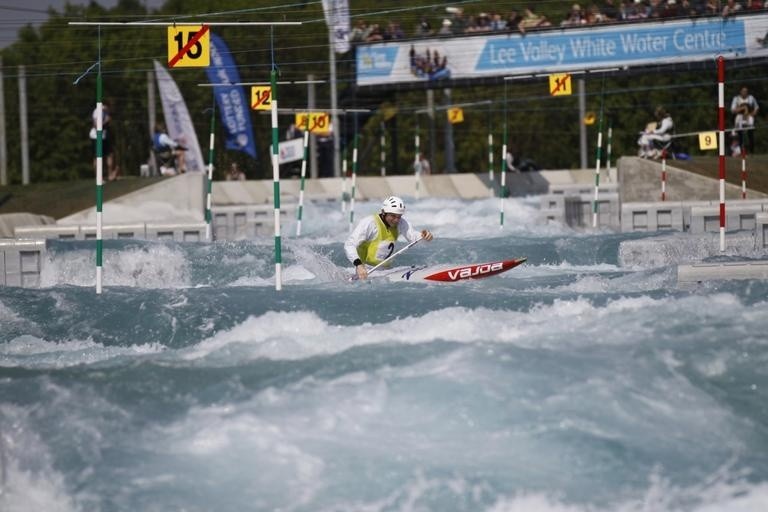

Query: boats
(273, 256), (527, 306)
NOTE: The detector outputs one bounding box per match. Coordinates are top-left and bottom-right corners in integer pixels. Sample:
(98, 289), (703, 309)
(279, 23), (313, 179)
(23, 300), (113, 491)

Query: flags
(208, 36), (255, 157)
(153, 61), (206, 174)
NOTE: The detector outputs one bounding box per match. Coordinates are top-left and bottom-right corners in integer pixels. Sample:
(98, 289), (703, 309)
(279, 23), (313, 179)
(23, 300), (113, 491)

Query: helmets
(381, 197), (405, 215)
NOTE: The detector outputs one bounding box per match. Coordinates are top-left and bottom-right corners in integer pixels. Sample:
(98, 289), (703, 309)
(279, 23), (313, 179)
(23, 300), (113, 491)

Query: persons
(639, 105), (676, 162)
(90, 98), (118, 180)
(224, 164), (246, 179)
(349, 2), (765, 44)
(730, 87), (759, 159)
(344, 196), (433, 282)
(413, 150), (431, 179)
(506, 149), (517, 172)
(152, 123), (186, 176)
